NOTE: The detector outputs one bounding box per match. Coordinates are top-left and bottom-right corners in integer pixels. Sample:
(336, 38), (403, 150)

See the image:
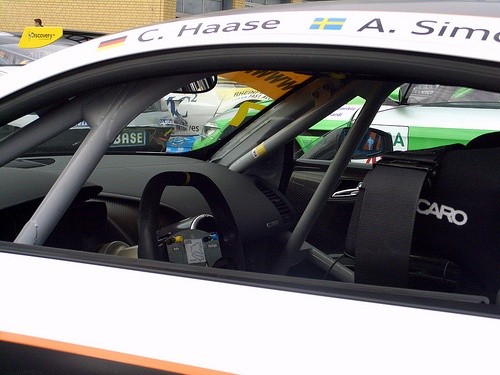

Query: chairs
(468, 132), (500, 175)
(347, 143), (500, 298)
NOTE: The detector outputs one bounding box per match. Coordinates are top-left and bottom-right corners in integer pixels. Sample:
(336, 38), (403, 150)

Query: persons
(34, 19), (43, 27)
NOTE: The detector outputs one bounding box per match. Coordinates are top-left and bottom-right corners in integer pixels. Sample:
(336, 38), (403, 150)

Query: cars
(0, 3), (500, 375)
(1, 34), (174, 133)
